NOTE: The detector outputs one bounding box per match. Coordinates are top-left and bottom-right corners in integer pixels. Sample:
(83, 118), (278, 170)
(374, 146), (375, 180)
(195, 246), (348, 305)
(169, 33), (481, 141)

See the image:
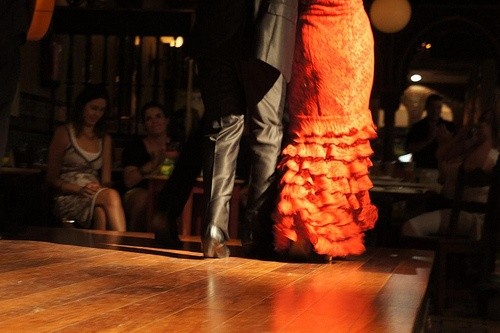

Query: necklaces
(84, 133), (93, 140)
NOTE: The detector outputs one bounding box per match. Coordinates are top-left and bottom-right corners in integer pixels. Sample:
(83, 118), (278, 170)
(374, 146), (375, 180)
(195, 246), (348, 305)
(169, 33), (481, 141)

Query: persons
(275, 0), (379, 262)
(46, 84), (127, 232)
(402, 123), (500, 242)
(183, 0), (299, 257)
(121, 104), (180, 233)
(404, 94), (458, 178)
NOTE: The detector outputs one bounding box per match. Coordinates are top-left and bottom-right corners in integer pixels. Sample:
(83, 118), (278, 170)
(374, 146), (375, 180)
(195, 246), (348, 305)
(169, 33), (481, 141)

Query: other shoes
(289, 239), (312, 263)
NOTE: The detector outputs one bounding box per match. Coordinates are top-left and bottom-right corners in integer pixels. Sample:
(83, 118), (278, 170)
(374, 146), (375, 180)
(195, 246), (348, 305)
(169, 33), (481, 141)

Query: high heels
(200, 223), (230, 258)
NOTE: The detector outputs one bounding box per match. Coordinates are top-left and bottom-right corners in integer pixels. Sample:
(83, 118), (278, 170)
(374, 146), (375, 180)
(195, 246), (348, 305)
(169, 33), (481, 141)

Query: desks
(139, 175), (241, 239)
(370, 177), (446, 249)
(0, 166), (40, 174)
